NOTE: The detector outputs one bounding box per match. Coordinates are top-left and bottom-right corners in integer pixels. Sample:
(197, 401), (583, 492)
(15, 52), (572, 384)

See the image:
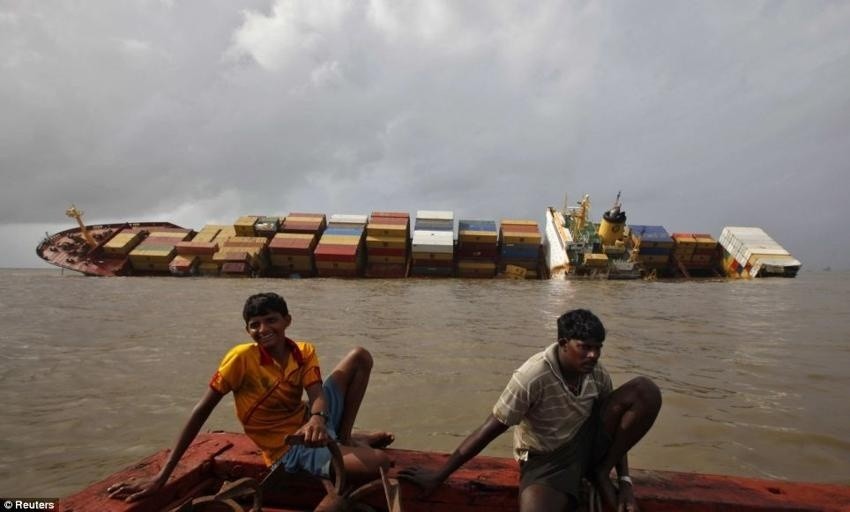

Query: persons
(107, 293), (394, 503)
(397, 309), (662, 512)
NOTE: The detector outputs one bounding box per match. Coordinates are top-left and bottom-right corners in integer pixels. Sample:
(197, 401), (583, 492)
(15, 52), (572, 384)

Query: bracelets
(310, 411), (330, 425)
(616, 476), (633, 487)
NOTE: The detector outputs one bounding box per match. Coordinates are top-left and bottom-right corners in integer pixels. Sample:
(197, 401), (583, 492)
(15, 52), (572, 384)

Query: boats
(35, 204), (800, 282)
